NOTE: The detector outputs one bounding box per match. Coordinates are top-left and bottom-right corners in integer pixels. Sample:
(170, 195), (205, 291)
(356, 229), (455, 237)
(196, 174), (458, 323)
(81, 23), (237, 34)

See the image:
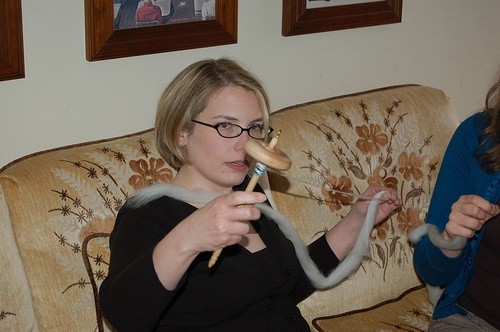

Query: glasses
(191, 119), (274, 140)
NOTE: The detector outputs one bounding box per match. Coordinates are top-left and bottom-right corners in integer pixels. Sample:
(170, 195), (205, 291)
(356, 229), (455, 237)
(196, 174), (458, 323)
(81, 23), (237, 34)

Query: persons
(413, 82), (500, 332)
(99, 59), (402, 332)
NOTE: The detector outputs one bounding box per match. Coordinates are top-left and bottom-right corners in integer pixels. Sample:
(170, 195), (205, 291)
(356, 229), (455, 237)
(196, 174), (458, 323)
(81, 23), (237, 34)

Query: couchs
(0, 83), (458, 332)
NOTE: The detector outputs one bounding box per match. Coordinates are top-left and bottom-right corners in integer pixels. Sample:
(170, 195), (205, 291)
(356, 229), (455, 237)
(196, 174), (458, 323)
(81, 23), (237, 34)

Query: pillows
(81, 233), (111, 332)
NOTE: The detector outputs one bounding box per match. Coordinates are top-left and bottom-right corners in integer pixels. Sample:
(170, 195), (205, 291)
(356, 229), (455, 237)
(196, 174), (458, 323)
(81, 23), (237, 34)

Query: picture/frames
(84, 0), (238, 62)
(282, 0), (403, 37)
